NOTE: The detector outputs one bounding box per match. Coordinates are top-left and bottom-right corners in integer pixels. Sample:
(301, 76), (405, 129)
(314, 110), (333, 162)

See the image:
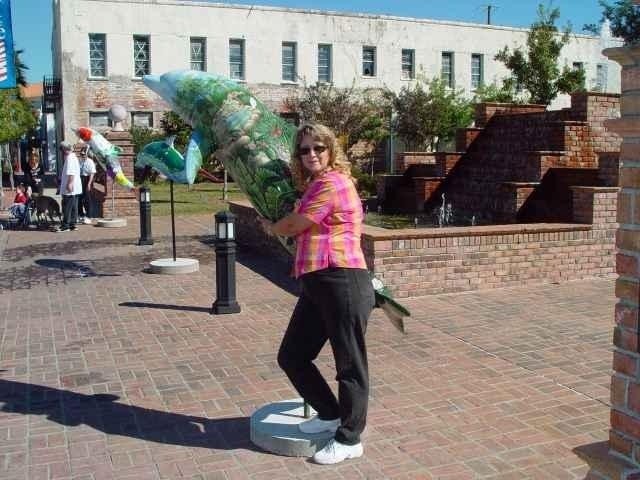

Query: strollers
(0, 182), (34, 231)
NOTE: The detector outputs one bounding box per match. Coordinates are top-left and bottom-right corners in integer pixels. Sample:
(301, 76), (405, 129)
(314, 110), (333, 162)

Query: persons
(12, 184), (28, 220)
(26, 147), (46, 225)
(254, 120), (378, 468)
(56, 141), (83, 233)
(79, 147), (97, 220)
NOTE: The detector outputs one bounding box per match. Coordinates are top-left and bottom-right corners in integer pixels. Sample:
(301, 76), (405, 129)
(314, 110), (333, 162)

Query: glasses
(298, 146), (328, 155)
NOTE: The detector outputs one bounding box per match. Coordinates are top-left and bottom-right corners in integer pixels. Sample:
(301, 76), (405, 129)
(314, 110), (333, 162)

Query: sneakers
(299, 415), (342, 434)
(313, 439), (363, 465)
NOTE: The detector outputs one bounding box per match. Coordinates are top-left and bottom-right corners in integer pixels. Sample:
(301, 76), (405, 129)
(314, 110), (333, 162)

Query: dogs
(31, 193), (62, 227)
